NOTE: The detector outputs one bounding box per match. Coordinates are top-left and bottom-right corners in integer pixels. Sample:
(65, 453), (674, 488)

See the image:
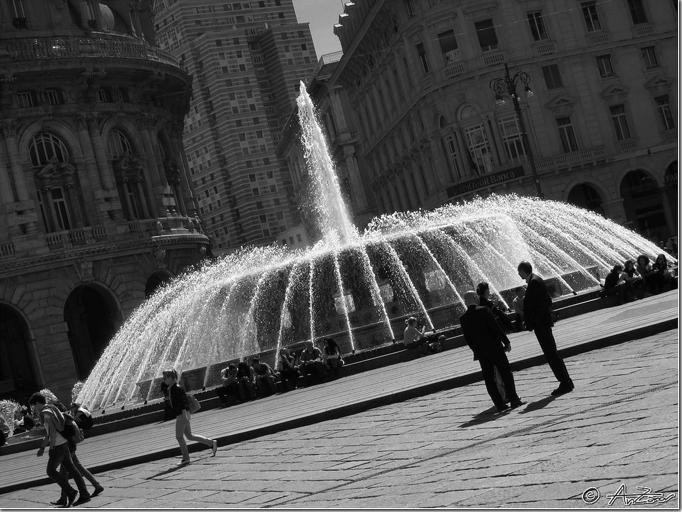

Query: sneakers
(212, 440), (217, 457)
(50, 485), (104, 507)
(177, 459), (190, 466)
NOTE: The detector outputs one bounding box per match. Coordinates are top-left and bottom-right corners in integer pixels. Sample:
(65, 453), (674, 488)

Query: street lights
(489, 61), (545, 198)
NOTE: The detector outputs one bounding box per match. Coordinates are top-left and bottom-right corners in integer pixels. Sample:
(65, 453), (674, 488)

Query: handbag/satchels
(67, 406), (93, 429)
(186, 394), (200, 413)
(43, 405), (81, 443)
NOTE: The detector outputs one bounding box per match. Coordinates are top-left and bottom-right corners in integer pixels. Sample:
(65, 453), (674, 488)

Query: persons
(459, 290), (527, 411)
(217, 338), (346, 407)
(12, 391), (103, 508)
(164, 371), (217, 466)
(518, 260), (574, 396)
(404, 317), (432, 357)
(513, 286), (525, 332)
(160, 370), (176, 421)
(477, 283), (521, 403)
(601, 254), (677, 304)
